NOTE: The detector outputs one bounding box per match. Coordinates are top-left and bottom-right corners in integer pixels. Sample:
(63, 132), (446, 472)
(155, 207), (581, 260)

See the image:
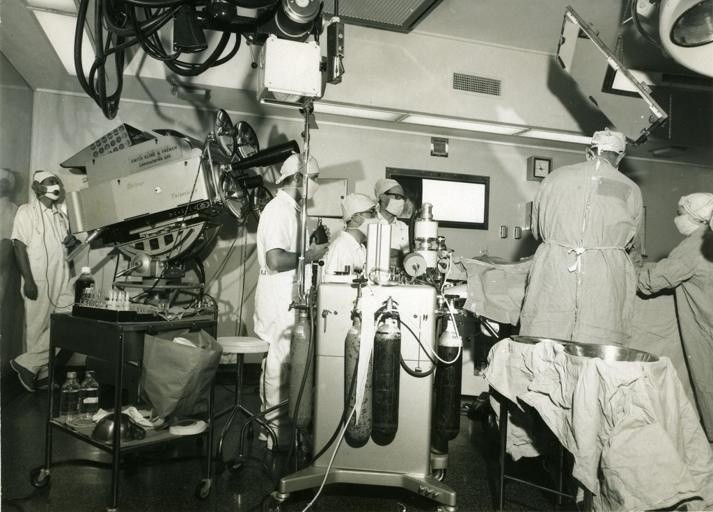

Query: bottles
(72, 266), (131, 313)
(57, 370), (100, 425)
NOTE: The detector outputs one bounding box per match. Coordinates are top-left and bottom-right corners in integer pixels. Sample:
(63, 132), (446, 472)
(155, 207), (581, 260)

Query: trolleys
(29, 313), (217, 512)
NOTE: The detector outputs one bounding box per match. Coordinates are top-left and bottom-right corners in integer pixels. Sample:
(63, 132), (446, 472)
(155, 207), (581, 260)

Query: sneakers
(9, 358), (59, 393)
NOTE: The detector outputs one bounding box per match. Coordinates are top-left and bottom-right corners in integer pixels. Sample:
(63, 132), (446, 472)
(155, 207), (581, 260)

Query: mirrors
(386, 167), (490, 230)
(555, 9), (661, 145)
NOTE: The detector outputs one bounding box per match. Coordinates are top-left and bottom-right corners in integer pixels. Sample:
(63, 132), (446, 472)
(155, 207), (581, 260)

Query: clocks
(527, 156), (553, 181)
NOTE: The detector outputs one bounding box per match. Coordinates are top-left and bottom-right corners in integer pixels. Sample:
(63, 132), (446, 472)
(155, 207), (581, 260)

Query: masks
(671, 214), (701, 236)
(45, 184), (61, 201)
(359, 215), (376, 236)
(384, 197), (405, 218)
(299, 175), (322, 201)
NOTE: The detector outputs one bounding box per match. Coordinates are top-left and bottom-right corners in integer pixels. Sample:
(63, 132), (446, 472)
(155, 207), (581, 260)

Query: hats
(679, 190), (713, 225)
(338, 192), (373, 223)
(32, 170), (57, 184)
(274, 154), (321, 185)
(373, 178), (399, 199)
(587, 125), (629, 156)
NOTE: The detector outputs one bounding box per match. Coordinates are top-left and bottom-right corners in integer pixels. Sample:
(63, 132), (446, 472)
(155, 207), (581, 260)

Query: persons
(629, 191), (712, 443)
(321, 191), (381, 284)
(372, 177), (411, 257)
(250, 154), (334, 452)
(0, 169), (24, 386)
(8, 170), (81, 393)
(517, 128), (643, 349)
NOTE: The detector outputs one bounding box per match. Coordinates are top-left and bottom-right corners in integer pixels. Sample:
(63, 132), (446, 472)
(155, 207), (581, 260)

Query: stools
(216, 336), (282, 464)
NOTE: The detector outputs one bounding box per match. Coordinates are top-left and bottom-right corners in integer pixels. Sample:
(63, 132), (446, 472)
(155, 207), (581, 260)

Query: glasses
(388, 190), (408, 200)
